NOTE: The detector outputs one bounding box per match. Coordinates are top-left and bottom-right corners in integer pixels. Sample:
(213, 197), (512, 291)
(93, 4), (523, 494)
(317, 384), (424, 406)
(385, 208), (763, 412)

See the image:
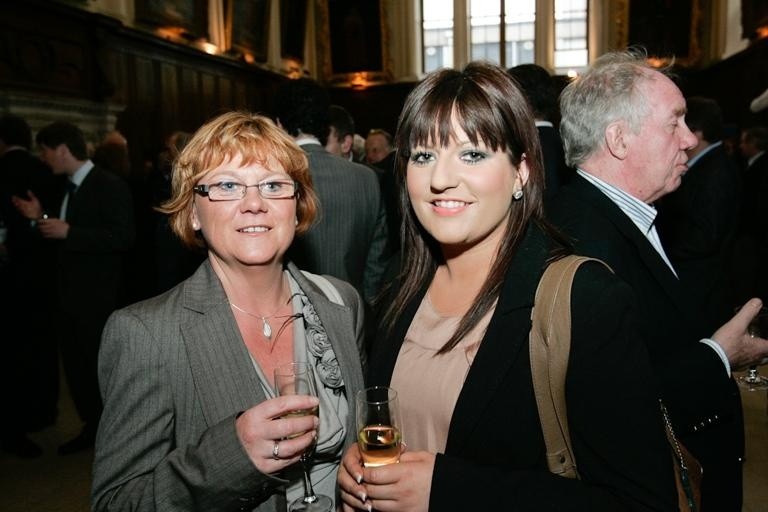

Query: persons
(1, 45), (767, 352)
(360, 57), (658, 512)
(89, 105), (373, 510)
(540, 48), (768, 510)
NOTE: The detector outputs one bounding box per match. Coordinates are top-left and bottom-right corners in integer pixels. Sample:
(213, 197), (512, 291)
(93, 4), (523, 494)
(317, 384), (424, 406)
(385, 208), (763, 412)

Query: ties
(65, 181), (76, 225)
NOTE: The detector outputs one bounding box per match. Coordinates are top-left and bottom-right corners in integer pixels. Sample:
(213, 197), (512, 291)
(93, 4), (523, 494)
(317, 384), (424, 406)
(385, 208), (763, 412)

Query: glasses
(188, 180), (299, 201)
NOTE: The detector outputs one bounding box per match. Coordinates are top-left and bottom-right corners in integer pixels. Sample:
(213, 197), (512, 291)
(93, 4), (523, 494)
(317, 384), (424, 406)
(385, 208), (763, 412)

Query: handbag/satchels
(662, 413), (705, 512)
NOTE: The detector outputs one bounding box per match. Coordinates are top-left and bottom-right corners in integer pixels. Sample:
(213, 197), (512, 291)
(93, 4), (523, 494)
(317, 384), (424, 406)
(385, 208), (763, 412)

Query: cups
(354, 385), (403, 467)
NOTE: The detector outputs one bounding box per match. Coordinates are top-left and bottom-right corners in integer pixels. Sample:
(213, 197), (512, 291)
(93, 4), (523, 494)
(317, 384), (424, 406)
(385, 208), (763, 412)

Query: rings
(268, 439), (282, 462)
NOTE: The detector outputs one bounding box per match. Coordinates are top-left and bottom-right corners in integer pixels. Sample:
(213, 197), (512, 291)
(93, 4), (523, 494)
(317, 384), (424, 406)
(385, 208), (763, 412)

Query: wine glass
(272, 362), (334, 511)
(731, 302), (768, 393)
